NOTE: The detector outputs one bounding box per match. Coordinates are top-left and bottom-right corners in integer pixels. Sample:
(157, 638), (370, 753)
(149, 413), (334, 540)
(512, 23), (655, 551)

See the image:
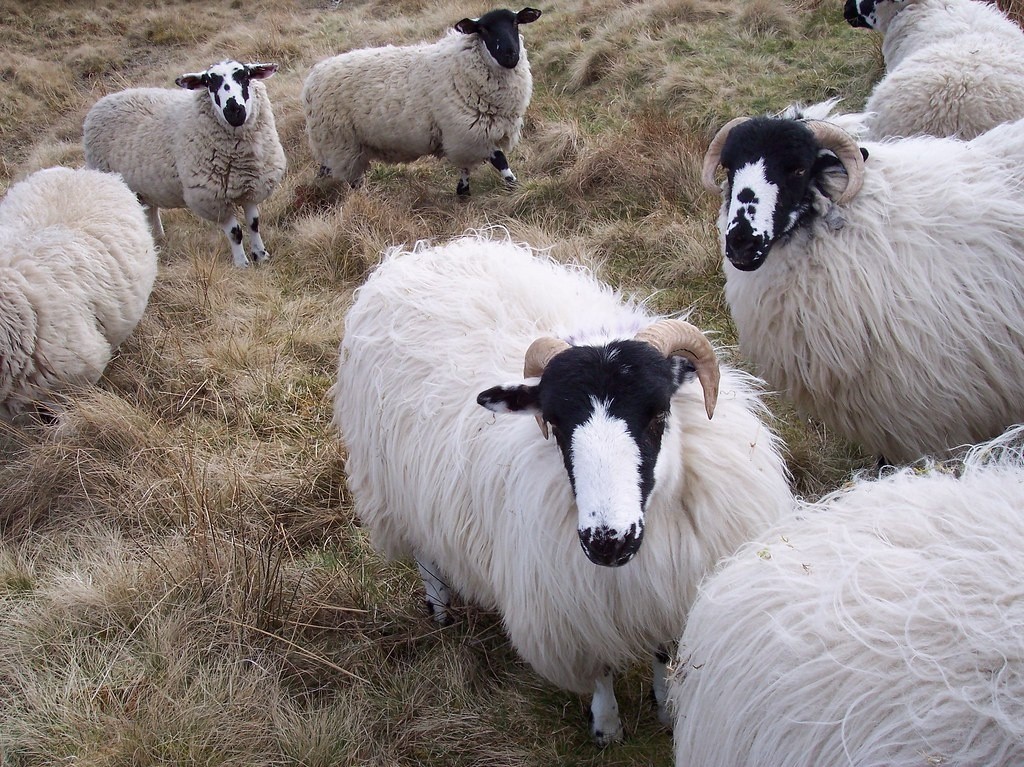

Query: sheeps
(0, 165), (155, 421)
(299, 7), (543, 201)
(81, 59), (288, 271)
(842, 0), (1024, 148)
(333, 224), (796, 750)
(699, 94), (1024, 470)
(668, 423), (1023, 767)
